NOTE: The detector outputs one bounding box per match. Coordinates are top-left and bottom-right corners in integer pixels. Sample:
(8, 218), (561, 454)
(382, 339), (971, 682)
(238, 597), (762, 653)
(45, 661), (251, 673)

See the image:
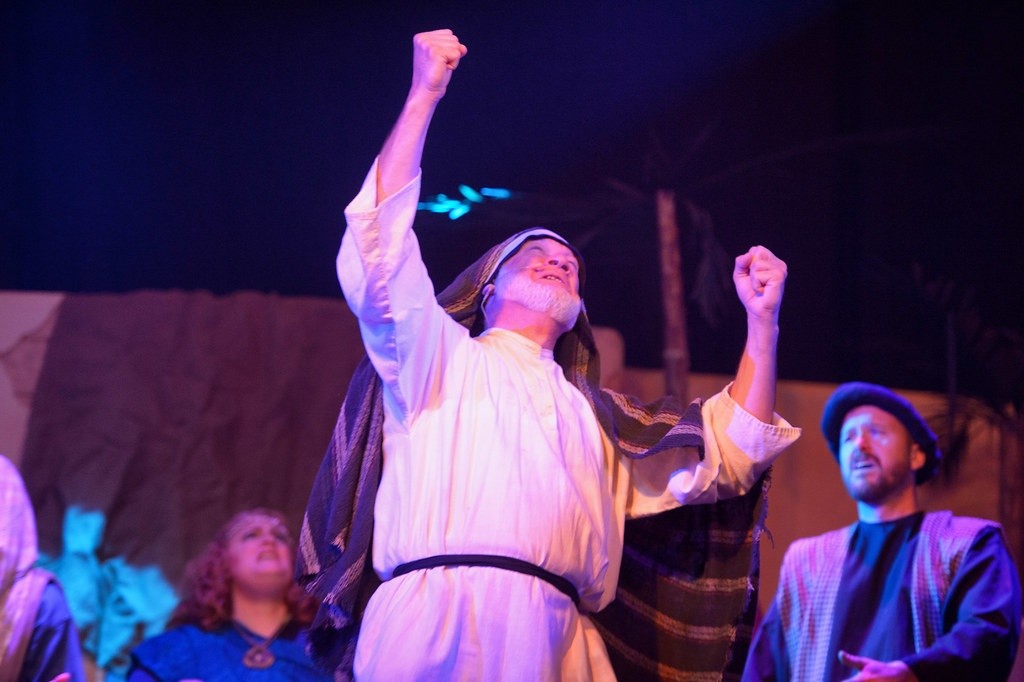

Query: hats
(820, 383), (941, 485)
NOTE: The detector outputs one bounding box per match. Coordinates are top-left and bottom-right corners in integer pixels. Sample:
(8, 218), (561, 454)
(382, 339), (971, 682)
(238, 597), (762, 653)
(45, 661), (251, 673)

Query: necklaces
(227, 622), (285, 670)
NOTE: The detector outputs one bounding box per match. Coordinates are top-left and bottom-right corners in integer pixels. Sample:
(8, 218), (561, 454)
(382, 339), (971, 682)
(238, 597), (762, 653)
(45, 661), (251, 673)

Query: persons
(739, 382), (1023, 682)
(296, 26), (803, 682)
(101, 506), (332, 680)
(0, 451), (88, 682)
